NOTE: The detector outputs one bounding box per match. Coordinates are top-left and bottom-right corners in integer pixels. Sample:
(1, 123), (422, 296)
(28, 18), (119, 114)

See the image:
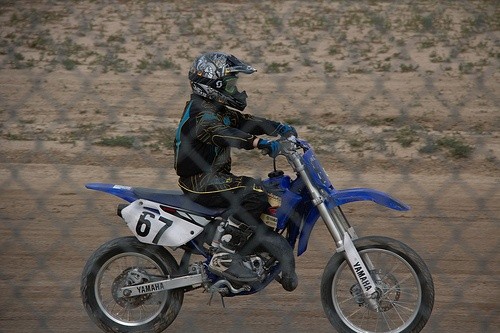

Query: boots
(208, 215), (257, 283)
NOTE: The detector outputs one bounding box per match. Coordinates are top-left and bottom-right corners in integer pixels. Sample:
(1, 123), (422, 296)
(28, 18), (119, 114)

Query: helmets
(187, 53), (258, 112)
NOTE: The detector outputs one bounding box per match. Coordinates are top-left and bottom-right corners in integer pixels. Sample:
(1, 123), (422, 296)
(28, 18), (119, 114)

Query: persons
(173, 50), (297, 285)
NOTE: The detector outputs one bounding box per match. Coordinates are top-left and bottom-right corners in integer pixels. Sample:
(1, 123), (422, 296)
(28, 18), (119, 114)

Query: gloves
(278, 123), (299, 142)
(259, 136), (280, 159)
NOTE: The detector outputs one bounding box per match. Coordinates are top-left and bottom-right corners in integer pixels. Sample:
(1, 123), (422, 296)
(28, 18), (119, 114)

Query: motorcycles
(80, 125), (435, 333)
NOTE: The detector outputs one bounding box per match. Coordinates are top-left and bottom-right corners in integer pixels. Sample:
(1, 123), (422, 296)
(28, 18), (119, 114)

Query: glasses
(224, 75), (237, 94)
